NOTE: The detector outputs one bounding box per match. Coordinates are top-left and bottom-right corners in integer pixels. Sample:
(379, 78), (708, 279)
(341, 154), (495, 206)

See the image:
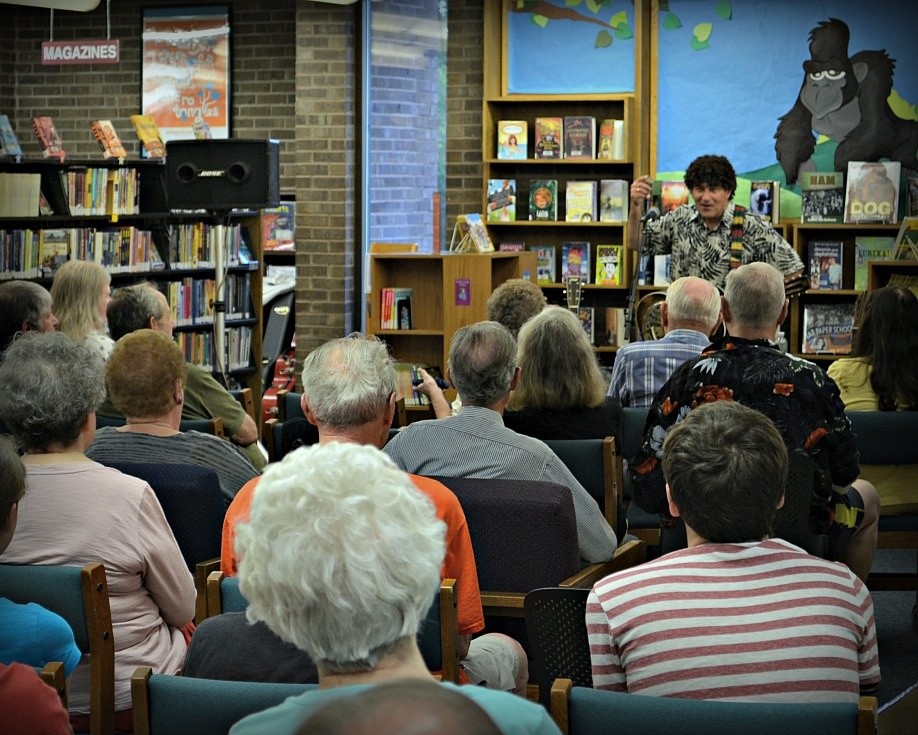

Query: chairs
(0, 386), (918, 735)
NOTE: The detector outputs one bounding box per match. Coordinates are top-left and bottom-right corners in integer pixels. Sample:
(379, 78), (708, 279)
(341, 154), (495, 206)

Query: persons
(225, 441), (559, 735)
(0, 261), (266, 735)
(507, 305), (626, 544)
(627, 155), (803, 295)
(220, 332), (528, 700)
(625, 262), (879, 582)
(828, 288), (918, 411)
(381, 320), (617, 562)
(412, 278), (546, 420)
(188, 611), (318, 684)
(610, 276), (724, 409)
(585, 401), (883, 703)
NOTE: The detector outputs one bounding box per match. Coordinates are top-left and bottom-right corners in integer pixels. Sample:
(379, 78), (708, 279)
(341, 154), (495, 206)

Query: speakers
(164, 139), (281, 210)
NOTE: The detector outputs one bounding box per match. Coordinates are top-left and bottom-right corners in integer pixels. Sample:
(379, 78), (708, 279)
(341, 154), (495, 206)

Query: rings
(637, 188), (638, 191)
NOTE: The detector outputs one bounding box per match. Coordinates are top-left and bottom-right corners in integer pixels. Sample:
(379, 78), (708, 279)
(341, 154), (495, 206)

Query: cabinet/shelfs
(366, 251), (537, 429)
(634, 222), (918, 361)
(0, 155), (263, 443)
(482, 94), (637, 353)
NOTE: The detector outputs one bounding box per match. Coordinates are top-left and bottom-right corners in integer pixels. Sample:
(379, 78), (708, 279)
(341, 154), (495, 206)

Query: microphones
(639, 207), (660, 223)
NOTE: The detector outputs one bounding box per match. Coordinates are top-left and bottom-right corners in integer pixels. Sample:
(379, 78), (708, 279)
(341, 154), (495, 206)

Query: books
(487, 116), (630, 345)
(750, 180), (780, 225)
(0, 114), (252, 368)
(638, 254), (672, 286)
(393, 363), (431, 406)
(380, 288), (413, 330)
(262, 203), (294, 251)
(802, 161), (918, 354)
(660, 180), (689, 216)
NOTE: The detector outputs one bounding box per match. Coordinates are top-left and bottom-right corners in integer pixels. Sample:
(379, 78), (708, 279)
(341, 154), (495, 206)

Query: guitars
(563, 276), (585, 316)
(635, 273), (812, 343)
(260, 331), (297, 428)
